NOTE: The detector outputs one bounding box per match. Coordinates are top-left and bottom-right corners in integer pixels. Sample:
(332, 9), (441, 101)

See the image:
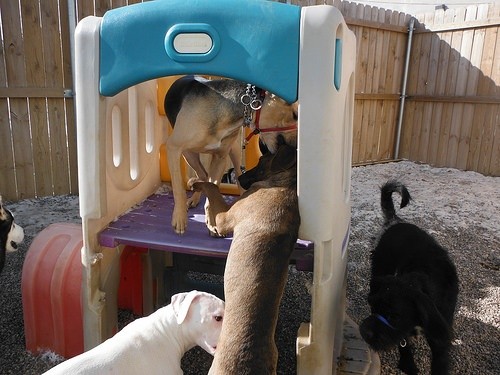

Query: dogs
(0, 201), (25, 258)
(40, 289), (225, 375)
(187, 144), (300, 375)
(359, 180), (459, 374)
(164, 75), (299, 237)
